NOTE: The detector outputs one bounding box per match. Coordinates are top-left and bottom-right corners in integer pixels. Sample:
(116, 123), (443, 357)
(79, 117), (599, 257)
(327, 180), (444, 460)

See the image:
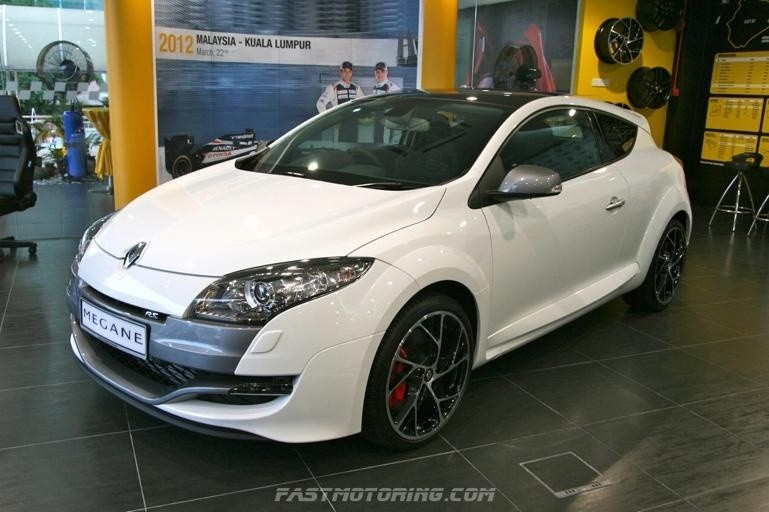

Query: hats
(340, 61), (353, 73)
(374, 62), (388, 71)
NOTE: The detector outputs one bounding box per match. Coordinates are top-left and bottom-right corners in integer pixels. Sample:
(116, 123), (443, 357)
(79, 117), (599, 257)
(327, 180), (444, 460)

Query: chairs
(746, 194), (769, 238)
(709, 151), (763, 234)
(0, 95), (39, 266)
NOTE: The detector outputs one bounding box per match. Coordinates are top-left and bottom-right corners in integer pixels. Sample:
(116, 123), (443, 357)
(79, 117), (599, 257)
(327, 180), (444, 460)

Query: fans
(35, 41), (94, 93)
(493, 42), (542, 91)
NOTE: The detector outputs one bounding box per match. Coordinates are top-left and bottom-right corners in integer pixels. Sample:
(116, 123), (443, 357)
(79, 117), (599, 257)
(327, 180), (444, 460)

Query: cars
(62, 88), (694, 457)
(164, 127), (258, 181)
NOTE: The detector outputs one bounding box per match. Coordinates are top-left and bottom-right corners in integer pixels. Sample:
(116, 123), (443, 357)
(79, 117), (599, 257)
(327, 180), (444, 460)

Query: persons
(315, 62), (366, 142)
(372, 62), (401, 144)
